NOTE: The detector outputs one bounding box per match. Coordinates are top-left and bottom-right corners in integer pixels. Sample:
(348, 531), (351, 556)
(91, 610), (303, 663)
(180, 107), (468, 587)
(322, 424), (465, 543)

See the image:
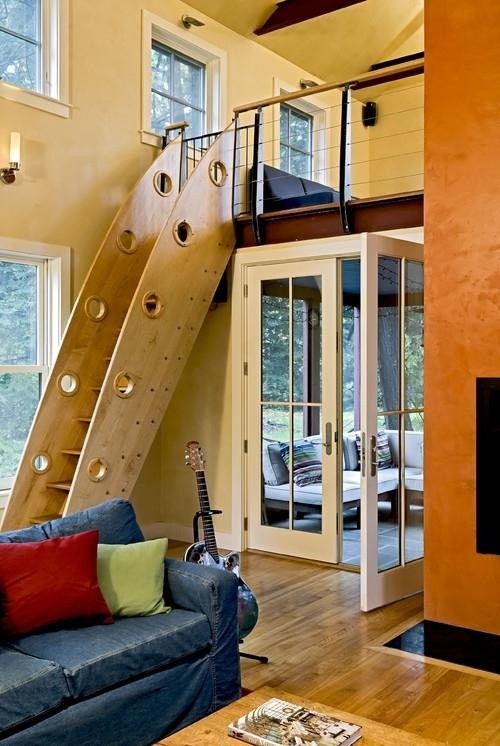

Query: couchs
(262, 427), (427, 536)
(250, 163), (340, 207)
(1, 496), (248, 746)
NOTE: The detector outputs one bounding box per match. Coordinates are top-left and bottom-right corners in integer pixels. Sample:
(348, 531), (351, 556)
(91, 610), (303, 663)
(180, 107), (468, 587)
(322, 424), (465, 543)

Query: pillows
(2, 533), (172, 636)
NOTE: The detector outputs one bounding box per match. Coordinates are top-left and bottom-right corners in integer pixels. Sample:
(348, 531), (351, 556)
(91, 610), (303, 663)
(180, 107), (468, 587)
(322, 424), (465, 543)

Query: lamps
(182, 13), (205, 30)
(3, 128), (22, 182)
(302, 80), (318, 90)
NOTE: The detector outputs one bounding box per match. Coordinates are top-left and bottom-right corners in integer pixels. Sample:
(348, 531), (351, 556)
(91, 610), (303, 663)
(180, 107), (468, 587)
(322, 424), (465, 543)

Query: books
(227, 697), (362, 746)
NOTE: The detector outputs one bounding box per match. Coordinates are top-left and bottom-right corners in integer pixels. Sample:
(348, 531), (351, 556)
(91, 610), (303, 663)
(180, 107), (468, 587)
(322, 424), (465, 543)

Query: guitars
(184, 441), (258, 644)
